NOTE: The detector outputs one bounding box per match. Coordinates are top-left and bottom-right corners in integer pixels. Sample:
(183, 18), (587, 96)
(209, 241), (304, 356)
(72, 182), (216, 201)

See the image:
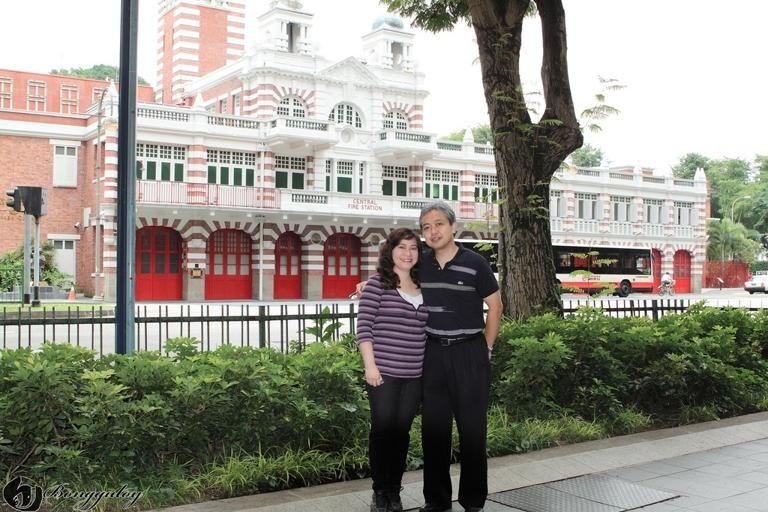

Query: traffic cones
(66, 285), (79, 301)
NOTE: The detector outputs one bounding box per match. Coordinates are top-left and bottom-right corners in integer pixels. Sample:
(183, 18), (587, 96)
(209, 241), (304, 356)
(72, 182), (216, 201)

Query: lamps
(137, 205), (421, 227)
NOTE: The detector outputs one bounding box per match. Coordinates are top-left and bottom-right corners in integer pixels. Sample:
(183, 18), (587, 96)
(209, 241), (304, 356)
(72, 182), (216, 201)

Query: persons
(660, 271), (673, 291)
(355, 226), (429, 511)
(353, 200), (505, 512)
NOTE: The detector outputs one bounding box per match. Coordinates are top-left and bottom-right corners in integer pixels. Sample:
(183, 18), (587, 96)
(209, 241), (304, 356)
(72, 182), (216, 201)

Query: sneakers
(371, 492), (403, 512)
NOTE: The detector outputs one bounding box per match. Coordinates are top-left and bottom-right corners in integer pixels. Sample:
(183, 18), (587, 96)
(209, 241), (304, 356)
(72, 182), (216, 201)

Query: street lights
(731, 195), (751, 224)
(254, 214), (267, 302)
(91, 82), (118, 307)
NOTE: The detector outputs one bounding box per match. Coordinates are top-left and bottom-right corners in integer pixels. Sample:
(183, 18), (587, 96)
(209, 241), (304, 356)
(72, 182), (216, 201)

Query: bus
(379, 237), (654, 299)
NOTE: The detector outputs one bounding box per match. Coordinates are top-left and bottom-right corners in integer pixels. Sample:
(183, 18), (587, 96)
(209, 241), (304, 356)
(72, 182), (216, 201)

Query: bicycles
(658, 280), (678, 297)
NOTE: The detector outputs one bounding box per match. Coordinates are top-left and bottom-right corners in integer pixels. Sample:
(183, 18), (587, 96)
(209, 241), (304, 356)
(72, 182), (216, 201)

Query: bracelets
(486, 342), (495, 353)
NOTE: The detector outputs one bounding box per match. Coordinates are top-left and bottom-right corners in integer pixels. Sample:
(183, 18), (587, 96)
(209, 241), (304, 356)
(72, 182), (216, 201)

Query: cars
(743, 274), (767, 293)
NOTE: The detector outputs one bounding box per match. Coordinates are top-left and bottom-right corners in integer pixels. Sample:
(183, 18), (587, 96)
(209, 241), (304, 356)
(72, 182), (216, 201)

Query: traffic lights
(4, 188), (21, 213)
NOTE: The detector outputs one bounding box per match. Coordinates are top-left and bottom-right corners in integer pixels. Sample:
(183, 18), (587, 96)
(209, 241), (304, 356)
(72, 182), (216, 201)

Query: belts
(437, 337), (469, 347)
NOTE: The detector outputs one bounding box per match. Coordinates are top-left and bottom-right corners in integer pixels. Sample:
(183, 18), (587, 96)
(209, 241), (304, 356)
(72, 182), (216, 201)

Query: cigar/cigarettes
(348, 288), (362, 298)
